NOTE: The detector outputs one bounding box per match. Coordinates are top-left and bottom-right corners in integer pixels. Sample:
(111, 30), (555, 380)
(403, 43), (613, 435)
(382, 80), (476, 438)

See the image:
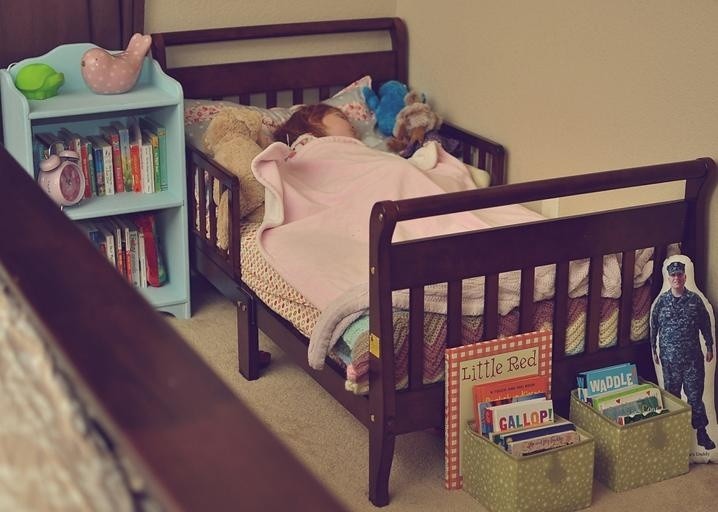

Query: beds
(148, 17), (717, 508)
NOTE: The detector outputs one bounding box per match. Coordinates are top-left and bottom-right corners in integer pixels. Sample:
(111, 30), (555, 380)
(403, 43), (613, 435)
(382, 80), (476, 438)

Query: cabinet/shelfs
(0, 42), (192, 321)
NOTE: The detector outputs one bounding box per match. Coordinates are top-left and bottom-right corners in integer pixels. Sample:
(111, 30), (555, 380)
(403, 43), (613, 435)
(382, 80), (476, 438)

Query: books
(441, 328), (554, 492)
(32, 114), (168, 293)
(473, 362), (670, 460)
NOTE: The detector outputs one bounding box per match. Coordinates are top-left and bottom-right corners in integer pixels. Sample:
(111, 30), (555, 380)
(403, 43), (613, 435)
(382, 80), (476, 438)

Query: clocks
(37, 142), (86, 212)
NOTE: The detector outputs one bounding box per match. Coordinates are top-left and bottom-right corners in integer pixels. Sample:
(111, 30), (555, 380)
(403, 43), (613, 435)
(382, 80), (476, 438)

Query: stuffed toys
(387, 88), (443, 159)
(14, 61), (65, 100)
(80, 31), (152, 95)
(361, 78), (410, 137)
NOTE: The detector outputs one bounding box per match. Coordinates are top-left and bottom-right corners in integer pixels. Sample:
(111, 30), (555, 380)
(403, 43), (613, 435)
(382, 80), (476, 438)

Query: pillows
(182, 75), (378, 156)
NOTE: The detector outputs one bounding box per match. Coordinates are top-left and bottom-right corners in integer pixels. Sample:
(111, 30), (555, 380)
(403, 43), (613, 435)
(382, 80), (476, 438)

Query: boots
(695, 426), (715, 451)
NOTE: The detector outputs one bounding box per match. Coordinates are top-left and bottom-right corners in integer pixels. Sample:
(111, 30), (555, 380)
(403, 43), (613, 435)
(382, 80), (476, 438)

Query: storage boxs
(570, 379), (693, 493)
(462, 415), (596, 512)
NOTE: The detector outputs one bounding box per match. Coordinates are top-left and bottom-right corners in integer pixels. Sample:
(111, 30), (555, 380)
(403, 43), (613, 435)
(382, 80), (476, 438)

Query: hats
(665, 261), (686, 277)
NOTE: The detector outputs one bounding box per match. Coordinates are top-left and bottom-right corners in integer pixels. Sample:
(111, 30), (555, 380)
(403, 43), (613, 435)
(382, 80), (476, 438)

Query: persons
(267, 101), (357, 160)
(647, 259), (716, 452)
